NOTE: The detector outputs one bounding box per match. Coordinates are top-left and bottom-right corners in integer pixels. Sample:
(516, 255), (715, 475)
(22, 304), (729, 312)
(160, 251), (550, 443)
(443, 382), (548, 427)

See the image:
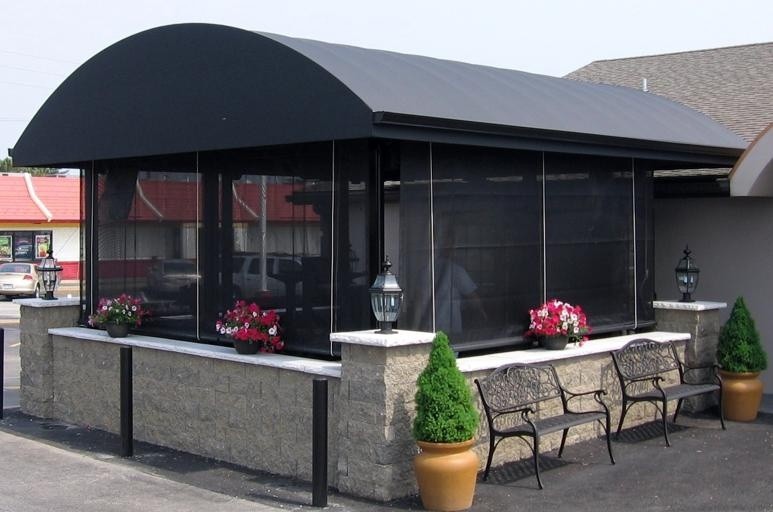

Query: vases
(539, 332), (568, 350)
(228, 337), (264, 355)
(102, 319), (132, 338)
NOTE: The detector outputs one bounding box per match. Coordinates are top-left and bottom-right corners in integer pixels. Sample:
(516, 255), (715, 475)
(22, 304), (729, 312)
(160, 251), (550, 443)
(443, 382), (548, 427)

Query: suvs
(214, 252), (306, 306)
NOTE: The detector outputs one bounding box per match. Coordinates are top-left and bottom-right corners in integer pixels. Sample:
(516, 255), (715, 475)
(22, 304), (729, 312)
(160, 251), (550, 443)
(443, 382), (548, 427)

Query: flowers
(523, 297), (592, 348)
(216, 299), (285, 355)
(84, 290), (152, 327)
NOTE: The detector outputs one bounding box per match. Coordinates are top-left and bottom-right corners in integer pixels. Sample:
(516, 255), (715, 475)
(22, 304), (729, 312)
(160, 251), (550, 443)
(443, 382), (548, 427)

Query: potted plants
(716, 293), (769, 426)
(405, 328), (481, 512)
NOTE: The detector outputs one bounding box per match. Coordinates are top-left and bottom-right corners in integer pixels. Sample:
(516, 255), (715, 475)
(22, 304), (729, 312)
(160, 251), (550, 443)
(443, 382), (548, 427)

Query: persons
(411, 235), (488, 358)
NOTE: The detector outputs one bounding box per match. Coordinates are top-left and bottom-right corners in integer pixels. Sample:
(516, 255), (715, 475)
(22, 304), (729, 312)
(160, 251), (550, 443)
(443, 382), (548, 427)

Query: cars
(146, 260), (200, 295)
(0, 261), (45, 301)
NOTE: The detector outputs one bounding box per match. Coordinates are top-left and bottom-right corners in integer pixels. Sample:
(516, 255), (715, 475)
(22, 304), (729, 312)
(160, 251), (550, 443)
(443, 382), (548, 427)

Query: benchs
(473, 361), (620, 492)
(609, 337), (726, 448)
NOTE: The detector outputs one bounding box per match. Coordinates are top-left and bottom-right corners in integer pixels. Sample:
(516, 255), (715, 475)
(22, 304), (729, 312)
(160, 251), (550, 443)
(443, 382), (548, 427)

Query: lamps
(367, 253), (403, 334)
(672, 244), (701, 302)
(34, 248), (65, 300)
